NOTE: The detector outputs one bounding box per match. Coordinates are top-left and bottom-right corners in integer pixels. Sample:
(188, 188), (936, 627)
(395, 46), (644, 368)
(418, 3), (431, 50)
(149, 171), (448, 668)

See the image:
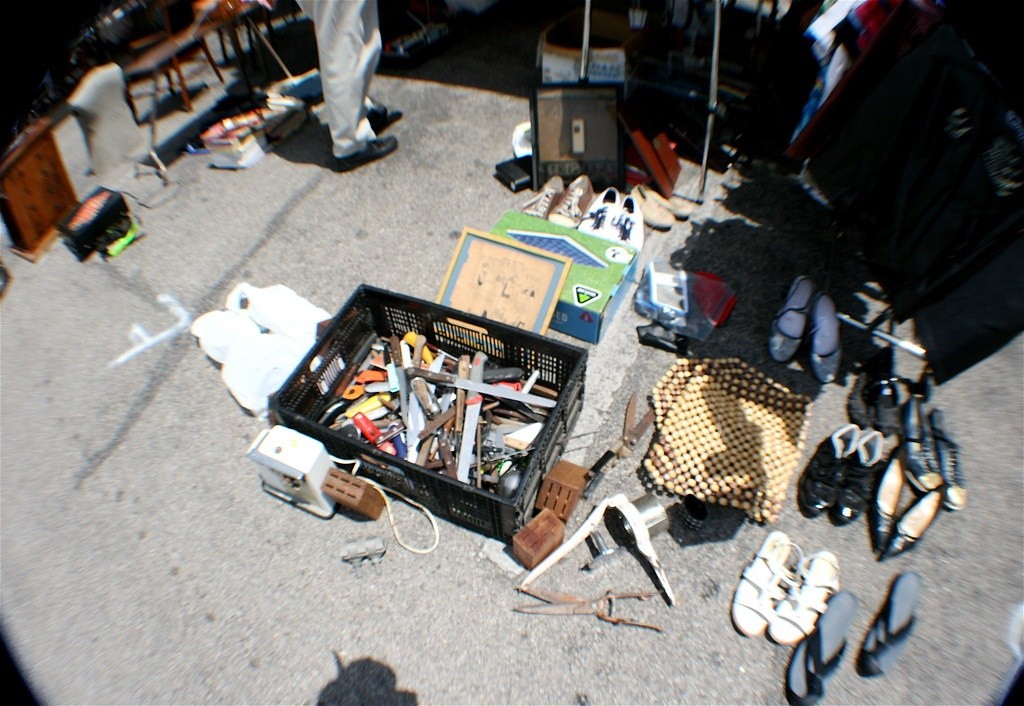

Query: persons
(296, 0), (403, 171)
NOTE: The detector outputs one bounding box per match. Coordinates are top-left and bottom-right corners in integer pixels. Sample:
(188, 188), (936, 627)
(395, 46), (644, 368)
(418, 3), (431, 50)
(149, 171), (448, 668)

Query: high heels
(877, 489), (943, 562)
(870, 456), (906, 552)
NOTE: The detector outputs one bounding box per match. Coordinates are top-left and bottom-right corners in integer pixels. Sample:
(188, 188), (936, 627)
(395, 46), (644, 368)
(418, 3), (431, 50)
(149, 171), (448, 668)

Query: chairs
(87, 1), (223, 123)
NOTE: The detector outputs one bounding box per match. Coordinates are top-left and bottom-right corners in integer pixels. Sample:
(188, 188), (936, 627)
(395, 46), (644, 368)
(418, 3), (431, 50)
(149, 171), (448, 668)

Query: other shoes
(769, 275), (818, 362)
(808, 292), (843, 384)
(645, 185), (691, 219)
(366, 108), (403, 135)
(330, 135), (398, 172)
(631, 185), (676, 230)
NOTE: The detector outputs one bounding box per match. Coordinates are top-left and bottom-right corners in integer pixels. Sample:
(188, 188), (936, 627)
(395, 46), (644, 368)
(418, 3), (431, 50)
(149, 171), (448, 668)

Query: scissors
(610, 393), (658, 460)
(513, 584), (665, 632)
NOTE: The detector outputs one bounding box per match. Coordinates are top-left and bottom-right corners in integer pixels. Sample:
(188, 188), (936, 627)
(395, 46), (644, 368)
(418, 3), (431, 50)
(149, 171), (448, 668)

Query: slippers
(785, 589), (859, 706)
(857, 571), (922, 677)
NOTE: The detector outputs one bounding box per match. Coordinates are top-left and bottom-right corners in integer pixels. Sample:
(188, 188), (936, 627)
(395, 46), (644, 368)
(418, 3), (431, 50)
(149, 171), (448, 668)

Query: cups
(610, 496), (670, 541)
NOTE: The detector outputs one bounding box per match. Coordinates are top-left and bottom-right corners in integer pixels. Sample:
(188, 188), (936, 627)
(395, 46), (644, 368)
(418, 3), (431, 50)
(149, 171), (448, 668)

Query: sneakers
(798, 424), (860, 519)
(828, 431), (882, 526)
(548, 174), (594, 228)
(577, 187), (620, 236)
(602, 196), (644, 252)
(927, 408), (966, 510)
(520, 175), (565, 220)
(900, 394), (944, 493)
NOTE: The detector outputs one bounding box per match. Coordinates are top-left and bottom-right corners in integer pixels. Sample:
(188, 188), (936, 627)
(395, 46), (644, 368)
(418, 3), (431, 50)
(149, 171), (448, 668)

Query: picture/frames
(435, 226), (574, 369)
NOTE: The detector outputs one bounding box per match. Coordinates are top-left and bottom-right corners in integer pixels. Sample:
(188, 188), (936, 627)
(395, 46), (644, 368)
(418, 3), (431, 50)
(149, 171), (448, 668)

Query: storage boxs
(387, 29), (452, 70)
(200, 67), (323, 168)
(271, 284), (589, 545)
(537, 8), (640, 102)
(489, 207), (640, 344)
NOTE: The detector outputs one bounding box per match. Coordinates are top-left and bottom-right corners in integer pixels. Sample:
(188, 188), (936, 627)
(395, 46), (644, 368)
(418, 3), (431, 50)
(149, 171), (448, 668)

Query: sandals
(768, 551), (840, 646)
(732, 531), (804, 637)
